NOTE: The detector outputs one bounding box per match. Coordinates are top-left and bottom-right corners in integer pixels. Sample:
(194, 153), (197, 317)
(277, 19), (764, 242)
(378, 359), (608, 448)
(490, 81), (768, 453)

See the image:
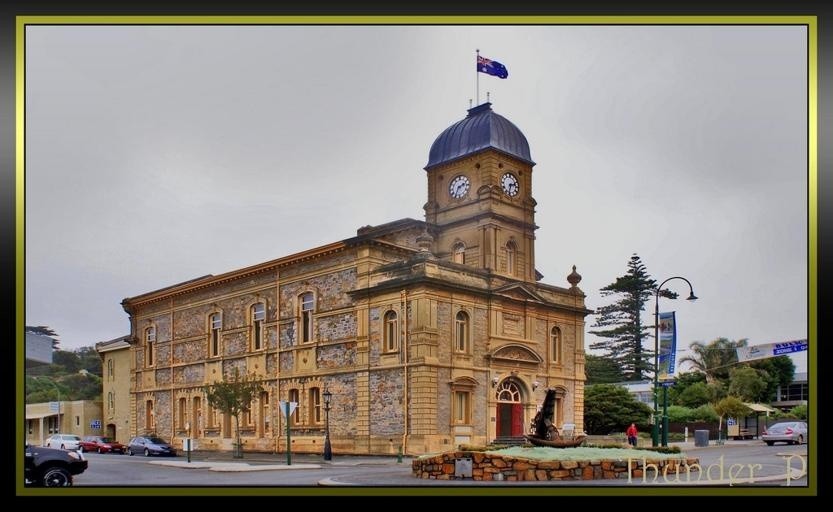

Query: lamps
(493, 377), (498, 386)
(533, 381), (539, 392)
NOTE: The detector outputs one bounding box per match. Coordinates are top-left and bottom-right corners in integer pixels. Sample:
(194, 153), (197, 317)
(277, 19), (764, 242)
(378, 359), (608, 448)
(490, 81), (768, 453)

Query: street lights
(652, 277), (698, 447)
(322, 389), (332, 461)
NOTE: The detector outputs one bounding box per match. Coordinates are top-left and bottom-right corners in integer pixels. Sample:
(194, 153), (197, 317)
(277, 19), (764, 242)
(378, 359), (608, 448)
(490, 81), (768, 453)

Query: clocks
(446, 172), (472, 203)
(498, 170), (523, 201)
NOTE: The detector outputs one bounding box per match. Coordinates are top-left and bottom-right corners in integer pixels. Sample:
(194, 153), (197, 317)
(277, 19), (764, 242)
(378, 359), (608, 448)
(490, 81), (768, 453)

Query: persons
(625, 422), (637, 445)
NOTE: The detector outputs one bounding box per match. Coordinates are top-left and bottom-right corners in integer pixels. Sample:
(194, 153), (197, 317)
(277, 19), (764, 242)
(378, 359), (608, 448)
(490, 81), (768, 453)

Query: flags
(475, 54), (508, 81)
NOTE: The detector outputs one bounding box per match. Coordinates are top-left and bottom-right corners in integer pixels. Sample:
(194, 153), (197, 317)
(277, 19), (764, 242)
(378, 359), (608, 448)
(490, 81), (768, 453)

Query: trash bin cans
(695, 430), (709, 446)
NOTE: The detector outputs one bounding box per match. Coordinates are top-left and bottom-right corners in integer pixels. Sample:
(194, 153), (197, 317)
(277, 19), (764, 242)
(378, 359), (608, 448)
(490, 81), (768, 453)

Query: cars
(762, 421), (807, 446)
(25, 443), (88, 487)
(126, 436), (177, 457)
(79, 436), (123, 454)
(46, 433), (82, 451)
